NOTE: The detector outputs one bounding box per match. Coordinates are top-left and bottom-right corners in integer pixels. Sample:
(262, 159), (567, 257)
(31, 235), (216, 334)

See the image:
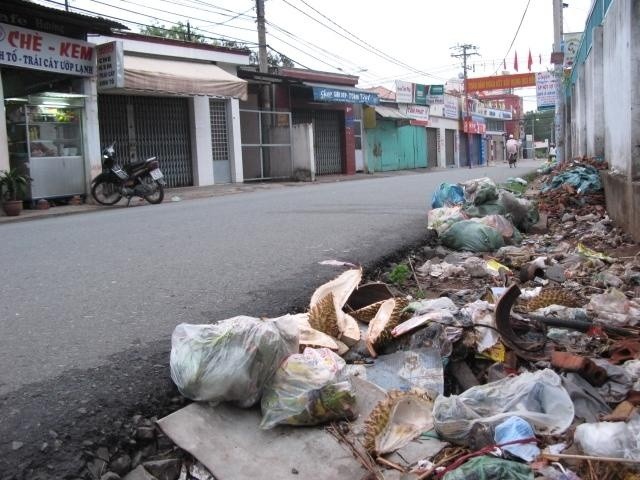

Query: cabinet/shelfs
(4, 93), (86, 207)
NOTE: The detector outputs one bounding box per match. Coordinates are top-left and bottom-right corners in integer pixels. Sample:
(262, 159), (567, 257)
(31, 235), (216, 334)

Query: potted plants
(0, 166), (35, 216)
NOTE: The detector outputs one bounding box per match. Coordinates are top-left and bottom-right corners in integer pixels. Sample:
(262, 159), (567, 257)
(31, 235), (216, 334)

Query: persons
(506, 134), (519, 168)
(548, 144), (556, 161)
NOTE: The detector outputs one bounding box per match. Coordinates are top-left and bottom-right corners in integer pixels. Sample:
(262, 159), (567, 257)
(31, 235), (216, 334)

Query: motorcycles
(90, 140), (166, 204)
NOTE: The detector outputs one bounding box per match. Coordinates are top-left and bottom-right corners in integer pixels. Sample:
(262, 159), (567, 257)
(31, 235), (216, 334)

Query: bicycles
(509, 153), (518, 169)
